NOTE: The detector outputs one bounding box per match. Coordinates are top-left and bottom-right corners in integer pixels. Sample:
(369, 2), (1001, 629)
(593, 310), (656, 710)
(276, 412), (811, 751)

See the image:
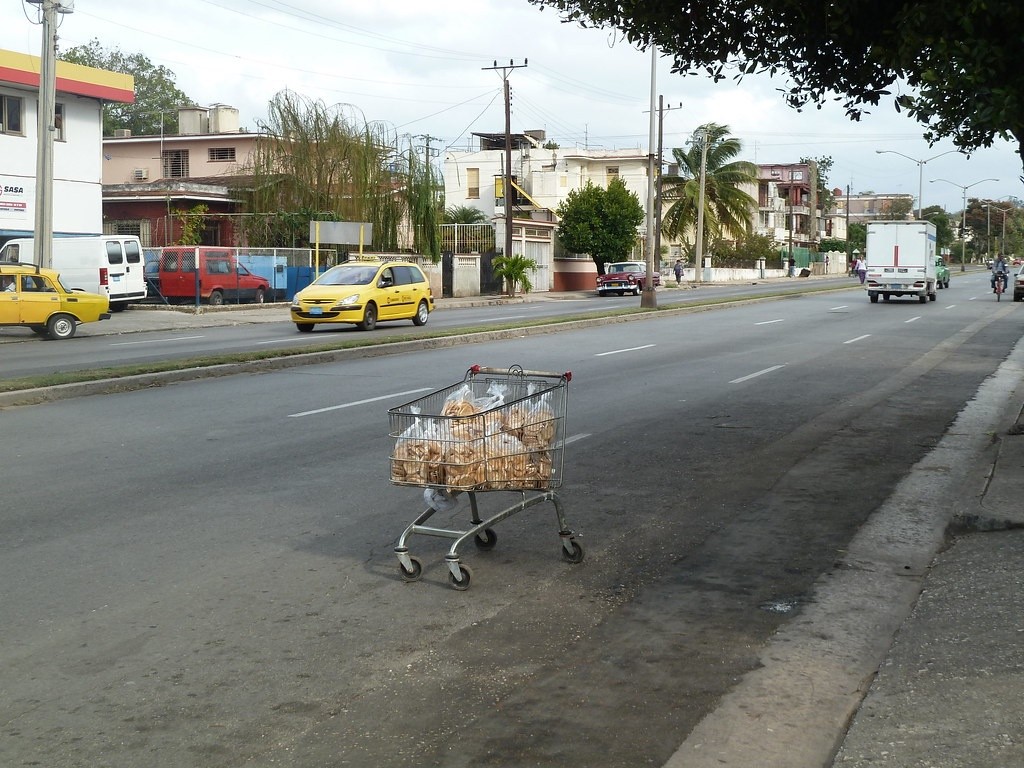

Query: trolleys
(388, 365), (586, 591)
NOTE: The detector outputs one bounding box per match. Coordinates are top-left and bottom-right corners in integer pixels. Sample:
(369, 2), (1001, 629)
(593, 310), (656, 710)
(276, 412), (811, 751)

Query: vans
(0, 234), (147, 312)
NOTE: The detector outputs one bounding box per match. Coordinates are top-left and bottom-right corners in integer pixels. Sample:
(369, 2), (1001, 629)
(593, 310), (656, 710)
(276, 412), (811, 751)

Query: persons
(4, 275), (16, 292)
(856, 256), (866, 284)
(990, 253), (1010, 293)
(673, 260), (684, 284)
(788, 256), (796, 277)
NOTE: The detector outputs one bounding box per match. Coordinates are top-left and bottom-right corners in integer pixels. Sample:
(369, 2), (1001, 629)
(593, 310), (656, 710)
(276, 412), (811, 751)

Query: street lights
(962, 194), (1024, 270)
(929, 178), (1000, 272)
(874, 150), (969, 219)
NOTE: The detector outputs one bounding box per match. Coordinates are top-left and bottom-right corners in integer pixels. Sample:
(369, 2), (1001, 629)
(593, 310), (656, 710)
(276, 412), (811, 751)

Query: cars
(1014, 262), (1024, 302)
(596, 262), (660, 295)
(290, 255), (435, 333)
(936, 255), (951, 289)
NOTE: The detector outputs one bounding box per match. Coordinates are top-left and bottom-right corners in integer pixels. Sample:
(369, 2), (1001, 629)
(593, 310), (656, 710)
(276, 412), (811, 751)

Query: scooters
(990, 264), (1010, 301)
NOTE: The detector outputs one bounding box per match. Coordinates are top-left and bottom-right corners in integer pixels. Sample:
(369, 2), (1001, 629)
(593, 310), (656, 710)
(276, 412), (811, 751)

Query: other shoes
(1002, 288), (1005, 292)
(994, 287), (997, 293)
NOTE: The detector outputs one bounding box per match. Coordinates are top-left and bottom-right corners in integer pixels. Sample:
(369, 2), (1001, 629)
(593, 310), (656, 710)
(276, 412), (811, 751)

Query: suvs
(0, 262), (111, 338)
(144, 258), (222, 297)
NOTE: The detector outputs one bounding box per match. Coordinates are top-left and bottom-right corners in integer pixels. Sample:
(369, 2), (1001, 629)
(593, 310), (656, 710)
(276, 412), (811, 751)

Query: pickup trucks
(159, 246), (269, 306)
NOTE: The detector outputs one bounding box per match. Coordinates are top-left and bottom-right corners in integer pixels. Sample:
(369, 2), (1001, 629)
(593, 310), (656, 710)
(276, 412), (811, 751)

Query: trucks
(865, 221), (939, 303)
